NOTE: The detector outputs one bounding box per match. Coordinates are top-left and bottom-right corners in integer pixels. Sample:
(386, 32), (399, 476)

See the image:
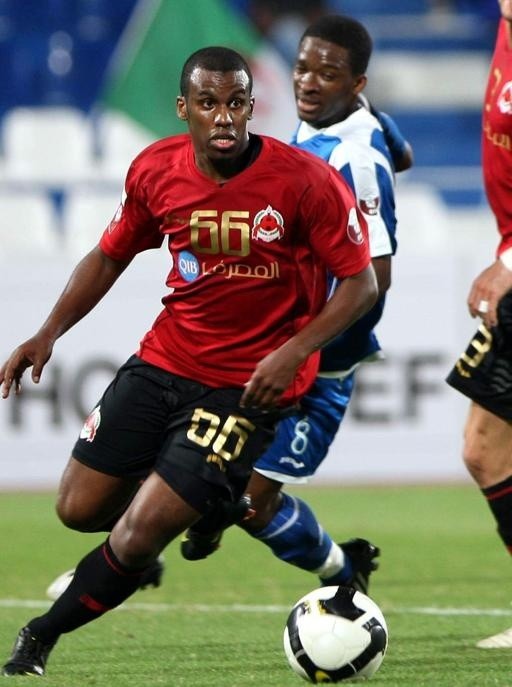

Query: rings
(478, 300), (489, 313)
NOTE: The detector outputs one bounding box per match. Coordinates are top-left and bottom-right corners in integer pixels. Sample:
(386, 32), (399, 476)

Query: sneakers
(319, 537), (381, 595)
(476, 628), (512, 648)
(47, 555), (163, 601)
(180, 494), (256, 561)
(3, 627), (56, 678)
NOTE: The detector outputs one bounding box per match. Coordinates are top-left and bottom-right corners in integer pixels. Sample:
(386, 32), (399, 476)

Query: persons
(445, 0), (512, 649)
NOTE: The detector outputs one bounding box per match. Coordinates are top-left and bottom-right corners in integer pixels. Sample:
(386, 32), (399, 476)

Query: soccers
(283, 585), (388, 684)
